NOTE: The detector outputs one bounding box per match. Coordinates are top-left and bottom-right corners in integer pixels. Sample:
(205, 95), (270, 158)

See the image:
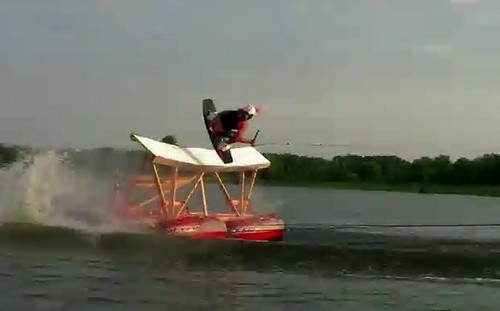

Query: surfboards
(201, 98), (235, 164)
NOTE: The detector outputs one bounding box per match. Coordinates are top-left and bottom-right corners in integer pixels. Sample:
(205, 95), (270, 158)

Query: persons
(210, 105), (262, 152)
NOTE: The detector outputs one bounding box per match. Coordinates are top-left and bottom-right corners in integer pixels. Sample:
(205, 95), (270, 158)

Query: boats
(117, 134), (286, 241)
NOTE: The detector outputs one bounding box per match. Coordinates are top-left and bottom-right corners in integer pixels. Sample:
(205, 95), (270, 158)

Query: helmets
(244, 105), (256, 120)
